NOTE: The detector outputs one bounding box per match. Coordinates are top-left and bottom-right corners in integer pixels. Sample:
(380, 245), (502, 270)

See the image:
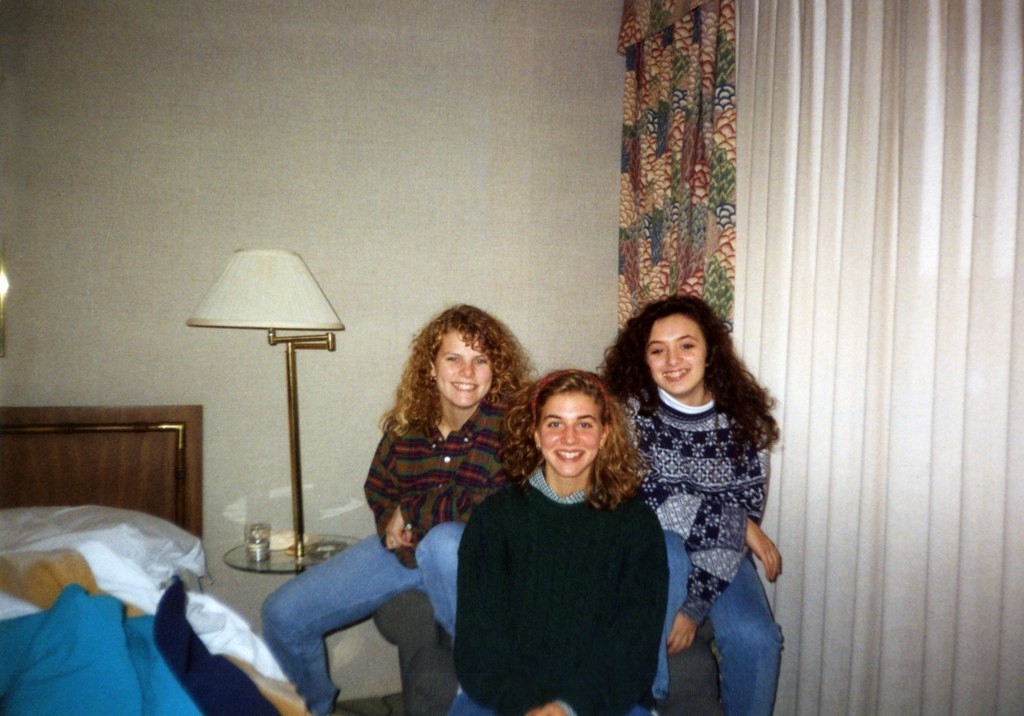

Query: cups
(244, 524), (271, 563)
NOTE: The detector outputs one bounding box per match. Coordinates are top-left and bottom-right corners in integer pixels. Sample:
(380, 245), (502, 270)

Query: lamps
(183, 247), (345, 559)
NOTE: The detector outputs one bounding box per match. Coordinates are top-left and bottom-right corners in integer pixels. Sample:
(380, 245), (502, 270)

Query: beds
(0, 403), (312, 716)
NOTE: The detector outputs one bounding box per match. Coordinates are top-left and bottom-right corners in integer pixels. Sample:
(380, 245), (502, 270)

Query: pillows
(0, 504), (207, 578)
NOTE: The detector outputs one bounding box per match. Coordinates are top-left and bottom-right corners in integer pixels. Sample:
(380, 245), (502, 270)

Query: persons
(262, 304), (535, 716)
(453, 370), (670, 716)
(600, 294), (785, 716)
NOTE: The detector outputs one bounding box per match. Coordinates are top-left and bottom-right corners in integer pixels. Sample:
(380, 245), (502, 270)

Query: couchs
(373, 590), (724, 716)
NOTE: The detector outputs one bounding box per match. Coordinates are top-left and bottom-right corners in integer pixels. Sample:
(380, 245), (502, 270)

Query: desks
(223, 533), (364, 577)
